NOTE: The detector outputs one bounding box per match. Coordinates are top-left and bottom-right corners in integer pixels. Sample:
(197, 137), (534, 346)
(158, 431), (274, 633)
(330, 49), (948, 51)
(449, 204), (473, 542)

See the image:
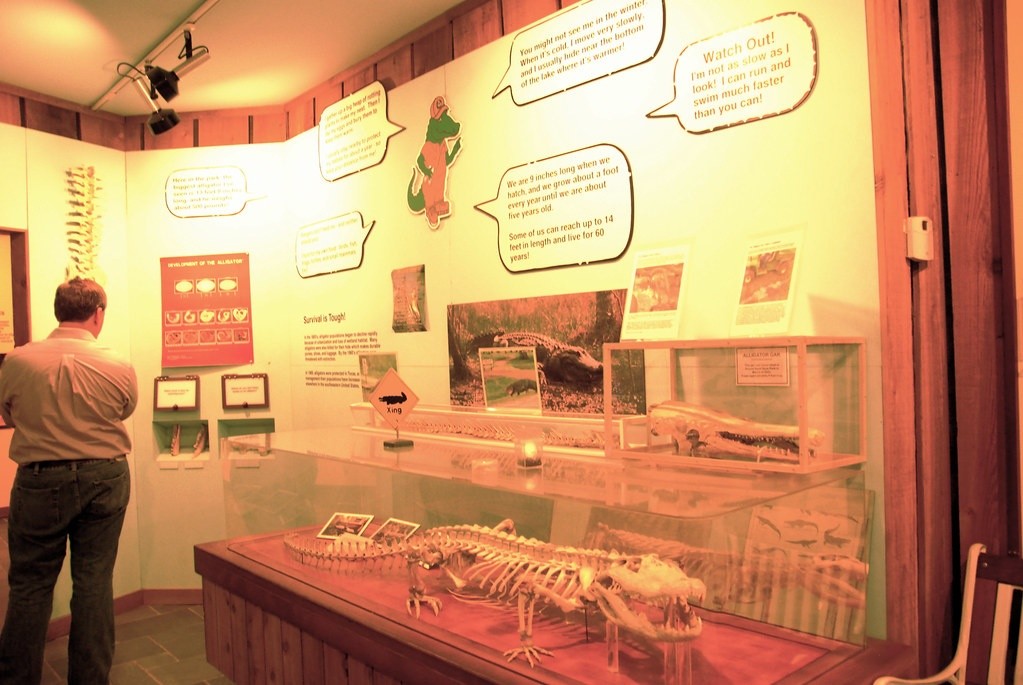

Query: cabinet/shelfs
(190, 522), (914, 685)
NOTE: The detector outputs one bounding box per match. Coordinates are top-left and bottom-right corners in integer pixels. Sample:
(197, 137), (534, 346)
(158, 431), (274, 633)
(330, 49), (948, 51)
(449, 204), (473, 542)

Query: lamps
(116, 23), (212, 134)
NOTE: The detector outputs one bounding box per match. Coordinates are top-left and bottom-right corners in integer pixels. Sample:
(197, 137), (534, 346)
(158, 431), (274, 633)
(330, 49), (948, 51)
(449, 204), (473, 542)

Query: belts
(30, 459), (110, 473)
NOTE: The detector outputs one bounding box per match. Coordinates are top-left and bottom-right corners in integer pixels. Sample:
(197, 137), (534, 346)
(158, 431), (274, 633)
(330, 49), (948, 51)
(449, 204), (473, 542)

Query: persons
(0, 277), (138, 685)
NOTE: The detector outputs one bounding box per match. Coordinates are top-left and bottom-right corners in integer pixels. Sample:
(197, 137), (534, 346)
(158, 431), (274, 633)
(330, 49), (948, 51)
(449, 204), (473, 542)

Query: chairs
(875, 544), (1023, 685)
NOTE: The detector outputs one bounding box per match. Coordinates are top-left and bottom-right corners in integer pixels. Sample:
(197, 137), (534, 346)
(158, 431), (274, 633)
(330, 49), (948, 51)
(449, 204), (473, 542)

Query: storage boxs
(597, 336), (868, 478)
(218, 423), (874, 685)
